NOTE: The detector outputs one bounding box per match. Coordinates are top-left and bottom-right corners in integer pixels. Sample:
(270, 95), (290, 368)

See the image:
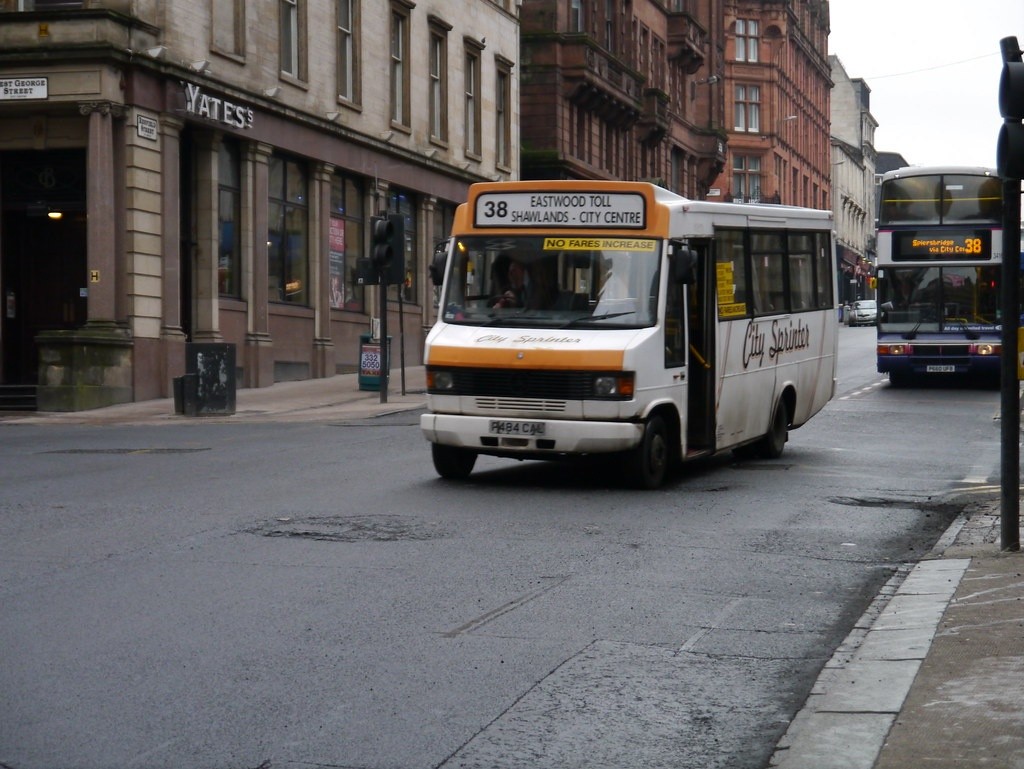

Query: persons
(483, 238), (545, 311)
(490, 262), (551, 314)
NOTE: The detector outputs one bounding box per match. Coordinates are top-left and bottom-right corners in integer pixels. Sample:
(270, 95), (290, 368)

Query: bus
(420, 178), (838, 489)
(877, 167), (1004, 387)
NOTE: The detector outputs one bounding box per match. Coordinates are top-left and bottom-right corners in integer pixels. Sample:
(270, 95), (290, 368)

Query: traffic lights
(997, 36), (1023, 178)
(367, 216), (395, 275)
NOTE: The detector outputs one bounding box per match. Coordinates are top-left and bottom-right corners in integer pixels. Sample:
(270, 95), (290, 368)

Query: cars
(848, 300), (877, 327)
